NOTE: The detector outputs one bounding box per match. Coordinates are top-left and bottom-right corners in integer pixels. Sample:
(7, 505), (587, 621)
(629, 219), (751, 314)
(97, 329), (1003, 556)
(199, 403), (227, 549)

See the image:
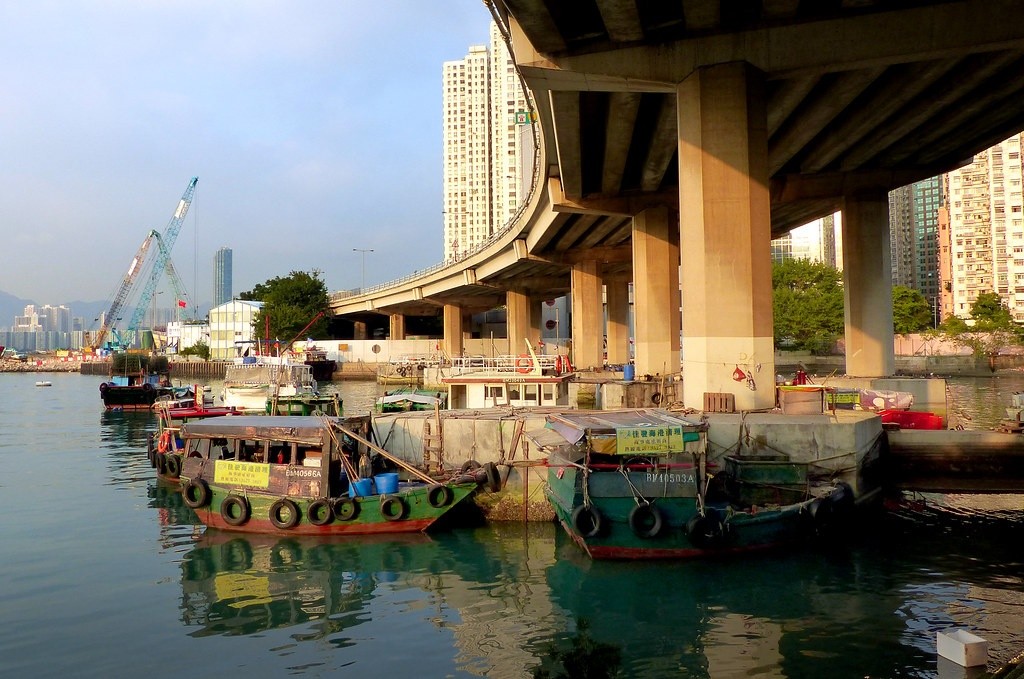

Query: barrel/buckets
(374, 473), (400, 494)
(169, 433), (184, 451)
(348, 478), (373, 498)
(243, 357), (256, 364)
(624, 364), (634, 381)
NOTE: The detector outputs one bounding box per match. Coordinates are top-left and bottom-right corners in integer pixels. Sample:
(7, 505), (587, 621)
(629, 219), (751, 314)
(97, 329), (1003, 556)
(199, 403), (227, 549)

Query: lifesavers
(556, 354), (562, 376)
(565, 355), (573, 373)
(157, 432), (171, 454)
(515, 353), (534, 374)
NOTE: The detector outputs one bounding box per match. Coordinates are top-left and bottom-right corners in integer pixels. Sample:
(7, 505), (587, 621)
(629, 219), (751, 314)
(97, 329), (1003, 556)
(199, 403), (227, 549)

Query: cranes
(83, 175), (200, 354)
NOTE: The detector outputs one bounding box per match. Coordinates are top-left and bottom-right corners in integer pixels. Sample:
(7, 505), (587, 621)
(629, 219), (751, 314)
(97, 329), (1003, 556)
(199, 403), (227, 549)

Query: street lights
(352, 248), (373, 289)
(506, 176), (523, 203)
(442, 211), (470, 255)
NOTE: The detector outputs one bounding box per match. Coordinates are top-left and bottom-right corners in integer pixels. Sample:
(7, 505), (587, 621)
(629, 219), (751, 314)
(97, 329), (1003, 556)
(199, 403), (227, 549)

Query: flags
(177, 299), (186, 308)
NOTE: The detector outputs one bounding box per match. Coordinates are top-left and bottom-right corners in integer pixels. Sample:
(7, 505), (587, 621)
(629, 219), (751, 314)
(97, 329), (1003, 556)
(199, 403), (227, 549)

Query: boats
(100, 374), (199, 413)
(374, 386), (448, 411)
(286, 346), (338, 380)
(218, 355), (318, 415)
(180, 416), (500, 535)
(445, 372), (576, 409)
(545, 413), (864, 560)
(146, 408), (245, 486)
(264, 390), (345, 417)
(36, 381), (52, 386)
